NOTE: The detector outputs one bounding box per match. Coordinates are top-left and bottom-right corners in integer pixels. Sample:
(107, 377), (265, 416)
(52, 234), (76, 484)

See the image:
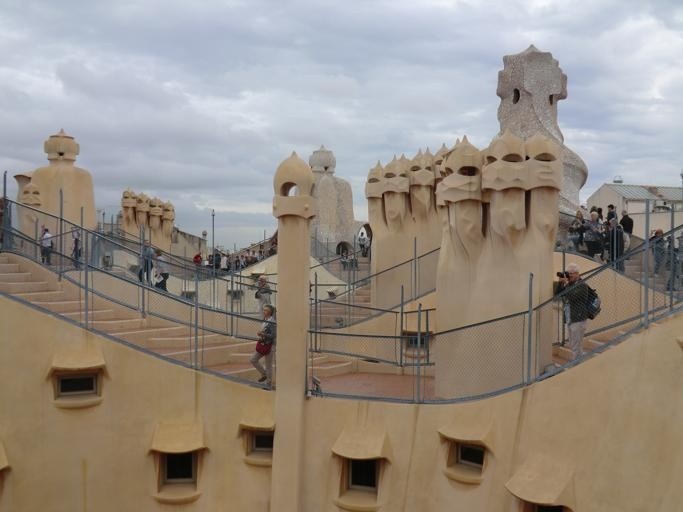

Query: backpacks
(622, 231), (630, 253)
(586, 286), (601, 320)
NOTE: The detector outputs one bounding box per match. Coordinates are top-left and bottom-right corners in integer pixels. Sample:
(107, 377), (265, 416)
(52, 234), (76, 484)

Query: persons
(40, 228), (53, 264)
(70, 236), (81, 271)
(152, 247), (169, 292)
(570, 203), (682, 291)
(137, 239), (153, 286)
(349, 250), (358, 267)
(253, 274), (272, 318)
(191, 242), (277, 279)
(357, 232), (367, 251)
(553, 262), (600, 361)
(249, 304), (276, 391)
(340, 250), (348, 270)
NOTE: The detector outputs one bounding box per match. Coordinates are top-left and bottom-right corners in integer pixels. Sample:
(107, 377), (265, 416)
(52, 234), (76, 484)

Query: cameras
(556, 271), (569, 279)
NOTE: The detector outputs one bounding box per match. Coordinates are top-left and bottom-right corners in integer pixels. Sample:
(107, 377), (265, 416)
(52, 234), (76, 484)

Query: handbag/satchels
(254, 290), (261, 299)
(255, 341), (272, 355)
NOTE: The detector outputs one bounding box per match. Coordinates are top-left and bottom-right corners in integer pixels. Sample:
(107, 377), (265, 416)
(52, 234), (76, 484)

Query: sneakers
(258, 375), (268, 382)
